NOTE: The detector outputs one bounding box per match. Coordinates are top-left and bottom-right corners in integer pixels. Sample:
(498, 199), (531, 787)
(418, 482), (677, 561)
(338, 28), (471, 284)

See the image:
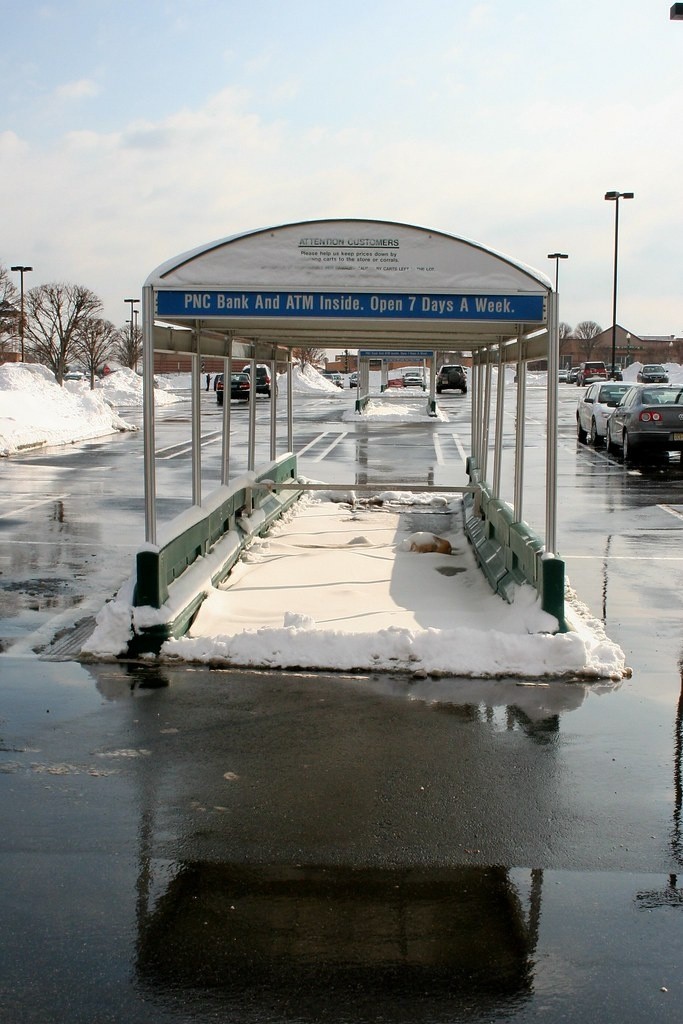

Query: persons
(206, 374), (212, 391)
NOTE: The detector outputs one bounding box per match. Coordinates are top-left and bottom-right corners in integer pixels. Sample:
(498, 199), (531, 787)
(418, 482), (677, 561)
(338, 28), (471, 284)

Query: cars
(559, 370), (568, 383)
(569, 366), (581, 384)
(323, 374), (344, 389)
(605, 366), (624, 382)
(637, 366), (670, 384)
(605, 383), (683, 459)
(213, 371), (250, 405)
(575, 381), (642, 446)
(63, 371), (101, 383)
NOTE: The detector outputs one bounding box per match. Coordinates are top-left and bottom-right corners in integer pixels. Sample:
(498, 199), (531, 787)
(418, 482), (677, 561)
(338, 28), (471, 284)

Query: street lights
(123, 298), (141, 373)
(10, 265), (34, 362)
(604, 190), (634, 382)
(547, 251), (569, 292)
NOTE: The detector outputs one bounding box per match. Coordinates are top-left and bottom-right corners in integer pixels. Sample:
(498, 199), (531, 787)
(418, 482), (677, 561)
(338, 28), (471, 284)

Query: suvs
(435, 364), (468, 393)
(242, 363), (279, 397)
(580, 361), (607, 387)
(349, 372), (360, 388)
(402, 372), (424, 387)
